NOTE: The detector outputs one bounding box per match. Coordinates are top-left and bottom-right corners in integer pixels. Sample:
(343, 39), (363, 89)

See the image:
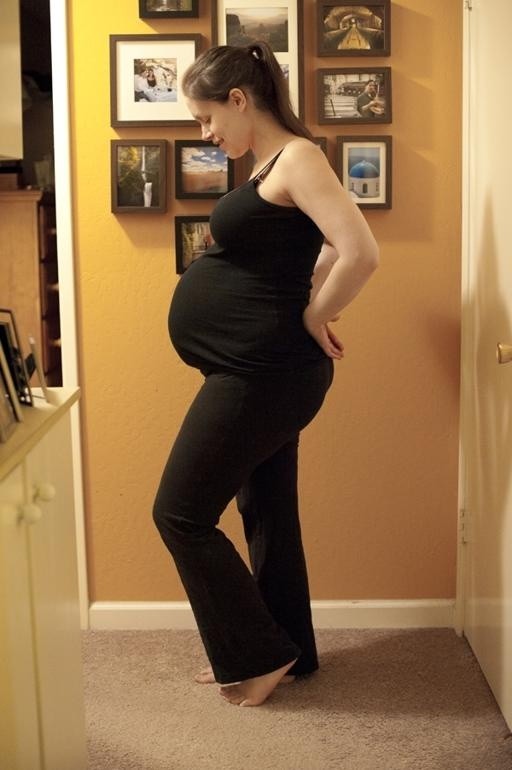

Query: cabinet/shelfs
(2, 388), (95, 770)
(0, 189), (62, 388)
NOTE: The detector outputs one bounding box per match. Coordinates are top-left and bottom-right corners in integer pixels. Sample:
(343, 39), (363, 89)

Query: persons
(353, 78), (386, 118)
(338, 15), (382, 50)
(146, 71), (161, 92)
(152, 42), (383, 710)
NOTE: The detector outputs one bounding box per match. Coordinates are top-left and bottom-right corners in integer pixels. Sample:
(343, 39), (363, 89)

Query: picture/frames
(336, 135), (393, 210)
(315, 136), (328, 152)
(315, 65), (393, 126)
(140, 1), (200, 19)
(109, 139), (167, 214)
(109, 31), (203, 127)
(315, 0), (392, 57)
(175, 214), (210, 273)
(174, 138), (234, 201)
(211, 2), (304, 125)
